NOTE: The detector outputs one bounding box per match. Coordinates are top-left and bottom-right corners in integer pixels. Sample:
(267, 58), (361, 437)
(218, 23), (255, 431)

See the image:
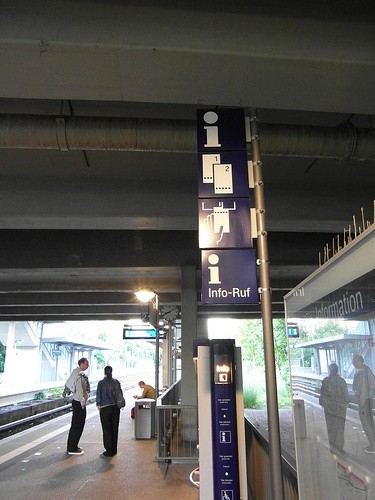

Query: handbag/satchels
(116, 380), (125, 408)
(131, 407), (135, 417)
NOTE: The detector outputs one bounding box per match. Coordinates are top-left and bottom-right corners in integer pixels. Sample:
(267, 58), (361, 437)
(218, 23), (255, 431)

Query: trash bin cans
(134, 398), (157, 440)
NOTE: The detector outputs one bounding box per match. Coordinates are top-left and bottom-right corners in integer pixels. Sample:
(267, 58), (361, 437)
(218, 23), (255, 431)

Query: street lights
(135, 285), (160, 399)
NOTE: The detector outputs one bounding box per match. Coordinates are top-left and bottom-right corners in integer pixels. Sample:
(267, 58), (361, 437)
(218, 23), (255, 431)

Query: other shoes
(99, 451), (113, 459)
(68, 448), (84, 455)
(364, 447), (375, 453)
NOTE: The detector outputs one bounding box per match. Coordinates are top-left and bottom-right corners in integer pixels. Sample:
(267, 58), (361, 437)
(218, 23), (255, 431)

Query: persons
(318, 355), (375, 456)
(67, 358), (90, 455)
(97, 366), (121, 457)
(133, 381), (159, 399)
